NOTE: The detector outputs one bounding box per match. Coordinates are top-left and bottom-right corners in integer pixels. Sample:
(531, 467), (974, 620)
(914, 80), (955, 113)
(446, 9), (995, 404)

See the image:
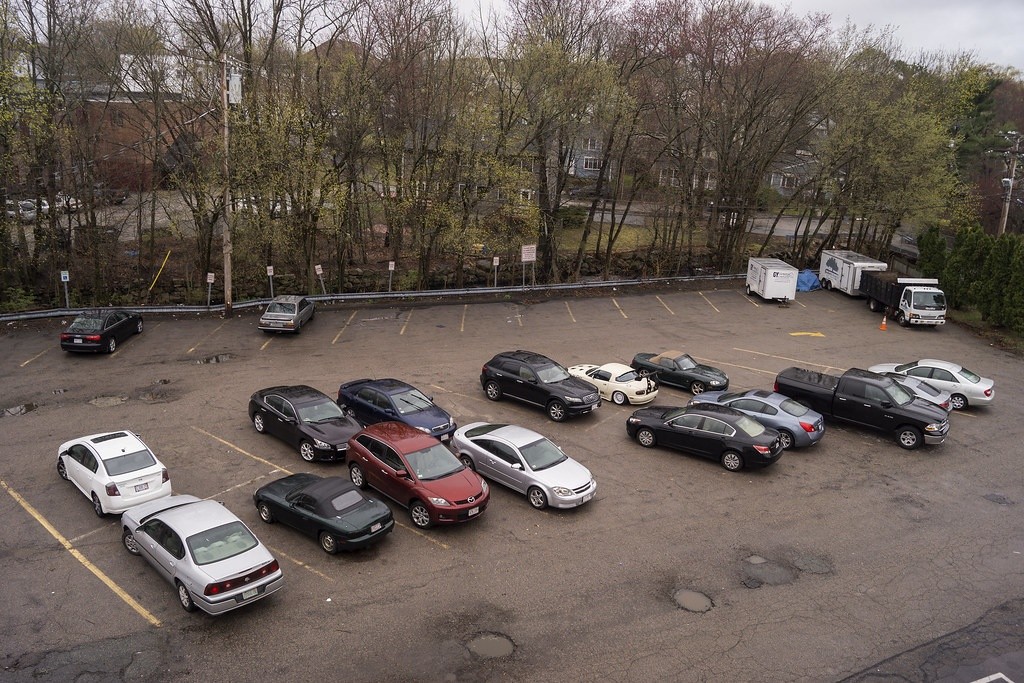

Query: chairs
(192, 533), (240, 553)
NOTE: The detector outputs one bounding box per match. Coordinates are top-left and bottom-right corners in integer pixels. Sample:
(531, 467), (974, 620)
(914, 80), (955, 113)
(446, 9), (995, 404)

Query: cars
(52, 195), (82, 213)
(25, 199), (49, 216)
(347, 421), (490, 529)
(60, 307), (143, 354)
(249, 385), (362, 464)
(254, 473), (396, 557)
(449, 421), (597, 510)
(121, 494), (284, 616)
(879, 372), (952, 414)
(57, 430), (173, 518)
(626, 403), (784, 472)
(631, 353), (729, 396)
(867, 359), (995, 411)
(566, 364), (659, 405)
(258, 295), (315, 335)
(337, 378), (457, 446)
(687, 388), (824, 450)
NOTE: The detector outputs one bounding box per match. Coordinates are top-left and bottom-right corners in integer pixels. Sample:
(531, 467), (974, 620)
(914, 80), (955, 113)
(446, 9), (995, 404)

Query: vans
(6, 199), (36, 223)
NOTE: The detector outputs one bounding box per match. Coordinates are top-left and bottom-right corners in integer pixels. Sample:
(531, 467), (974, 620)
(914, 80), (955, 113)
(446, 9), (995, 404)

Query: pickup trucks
(774, 367), (951, 450)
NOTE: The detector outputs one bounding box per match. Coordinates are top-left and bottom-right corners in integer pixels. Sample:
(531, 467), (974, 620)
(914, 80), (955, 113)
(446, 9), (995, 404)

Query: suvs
(480, 350), (602, 423)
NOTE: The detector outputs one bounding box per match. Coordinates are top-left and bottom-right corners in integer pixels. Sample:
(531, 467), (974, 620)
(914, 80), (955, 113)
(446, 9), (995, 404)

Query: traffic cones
(880, 315), (888, 331)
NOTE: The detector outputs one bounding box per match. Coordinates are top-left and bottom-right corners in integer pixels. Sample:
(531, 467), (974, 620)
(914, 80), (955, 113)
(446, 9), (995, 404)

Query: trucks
(859, 270), (946, 329)
